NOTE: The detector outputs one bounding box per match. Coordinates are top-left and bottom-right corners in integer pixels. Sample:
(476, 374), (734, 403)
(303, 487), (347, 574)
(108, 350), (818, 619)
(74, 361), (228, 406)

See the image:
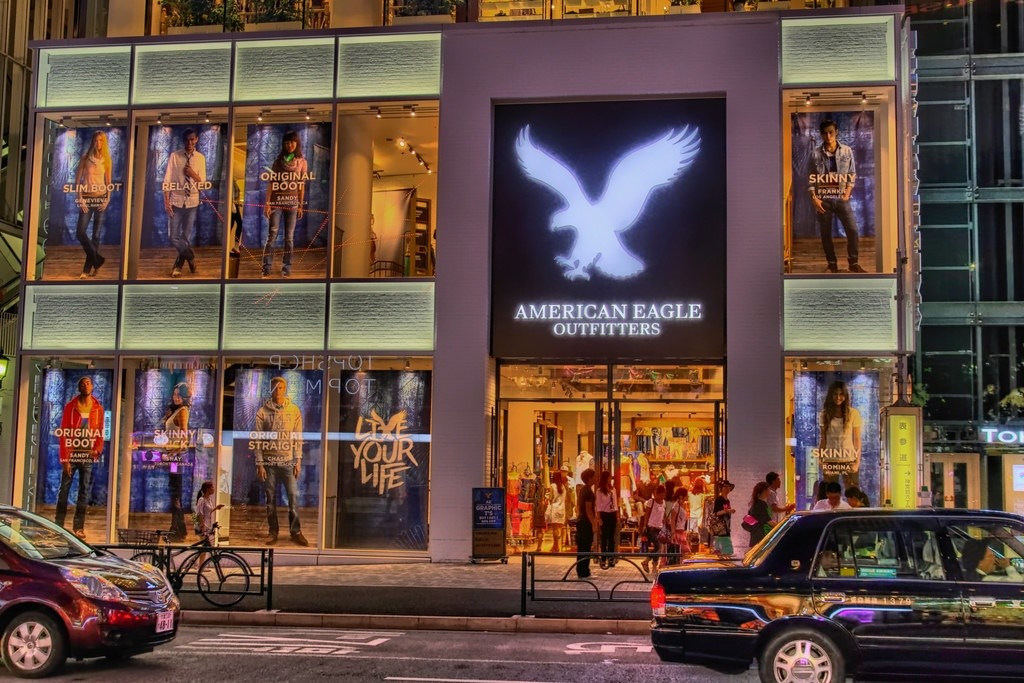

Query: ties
(183, 153), (194, 198)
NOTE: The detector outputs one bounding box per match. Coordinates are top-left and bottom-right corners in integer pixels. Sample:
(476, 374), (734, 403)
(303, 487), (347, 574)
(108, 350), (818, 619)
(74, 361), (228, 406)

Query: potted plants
(158, 0), (465, 35)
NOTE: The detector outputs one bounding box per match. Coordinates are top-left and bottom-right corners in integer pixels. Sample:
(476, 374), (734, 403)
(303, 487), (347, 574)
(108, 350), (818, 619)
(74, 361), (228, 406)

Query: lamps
(156, 117), (162, 124)
(806, 94), (869, 104)
(400, 138), (432, 174)
(306, 112), (310, 119)
(204, 115), (210, 123)
(411, 108), (416, 116)
(405, 360), (410, 369)
(376, 110), (382, 118)
(258, 113), (263, 121)
(90, 359), (96, 367)
(58, 119), (64, 127)
(105, 118), (112, 126)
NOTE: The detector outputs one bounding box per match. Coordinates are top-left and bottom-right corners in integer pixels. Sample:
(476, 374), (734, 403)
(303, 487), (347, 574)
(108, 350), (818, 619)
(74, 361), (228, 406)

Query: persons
(253, 377), (308, 546)
(192, 482), (223, 572)
(808, 120), (867, 273)
(748, 472), (787, 548)
(55, 378), (104, 537)
(821, 542), (836, 569)
(508, 467), (536, 515)
(818, 381), (863, 489)
(161, 382), (191, 540)
(575, 469), (598, 579)
(620, 453), (637, 517)
(712, 480), (735, 554)
(76, 130), (112, 279)
(961, 540), (1024, 582)
(813, 481), (869, 510)
(549, 465), (573, 552)
(596, 470), (620, 566)
(163, 129), (207, 278)
(262, 131), (308, 275)
(641, 477), (706, 573)
(575, 451), (595, 485)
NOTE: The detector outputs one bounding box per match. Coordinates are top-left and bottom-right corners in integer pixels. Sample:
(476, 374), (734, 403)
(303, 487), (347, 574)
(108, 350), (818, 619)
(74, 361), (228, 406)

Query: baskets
(117, 528), (160, 544)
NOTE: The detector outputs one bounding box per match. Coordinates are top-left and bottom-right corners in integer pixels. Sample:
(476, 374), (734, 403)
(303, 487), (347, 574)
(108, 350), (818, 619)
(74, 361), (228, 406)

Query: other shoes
(550, 547), (559, 552)
(75, 528), (86, 540)
(579, 575), (598, 580)
(824, 261), (837, 275)
(80, 272), (89, 279)
(172, 267), (182, 278)
(163, 532), (186, 543)
(91, 266), (101, 277)
(188, 247), (196, 273)
(291, 532), (308, 547)
(266, 532), (278, 545)
(282, 263), (291, 277)
(641, 562), (650, 573)
(602, 561), (606, 566)
(850, 262), (867, 274)
(263, 268), (272, 277)
(608, 559), (615, 567)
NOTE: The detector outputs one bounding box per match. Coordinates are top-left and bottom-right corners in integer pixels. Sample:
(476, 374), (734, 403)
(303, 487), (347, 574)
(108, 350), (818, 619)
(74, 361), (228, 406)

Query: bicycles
(118, 520), (250, 607)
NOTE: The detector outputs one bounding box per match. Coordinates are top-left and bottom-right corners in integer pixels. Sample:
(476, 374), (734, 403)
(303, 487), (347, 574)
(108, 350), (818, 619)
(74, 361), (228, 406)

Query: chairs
(921, 536), (956, 580)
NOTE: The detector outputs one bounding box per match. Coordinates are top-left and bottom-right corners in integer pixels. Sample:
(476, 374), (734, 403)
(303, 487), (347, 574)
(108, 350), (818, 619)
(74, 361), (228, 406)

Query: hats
(719, 480), (735, 492)
(560, 466), (569, 472)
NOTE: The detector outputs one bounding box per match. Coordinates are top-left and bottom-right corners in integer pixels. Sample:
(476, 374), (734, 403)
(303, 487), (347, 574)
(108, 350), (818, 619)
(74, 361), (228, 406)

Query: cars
(0, 503), (182, 680)
(649, 507), (1024, 683)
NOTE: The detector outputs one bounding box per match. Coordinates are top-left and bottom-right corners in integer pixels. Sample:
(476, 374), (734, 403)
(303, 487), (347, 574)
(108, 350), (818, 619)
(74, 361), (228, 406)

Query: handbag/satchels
(194, 526), (203, 534)
(708, 518), (725, 535)
(665, 521), (677, 533)
(740, 514), (758, 531)
(657, 524), (673, 545)
